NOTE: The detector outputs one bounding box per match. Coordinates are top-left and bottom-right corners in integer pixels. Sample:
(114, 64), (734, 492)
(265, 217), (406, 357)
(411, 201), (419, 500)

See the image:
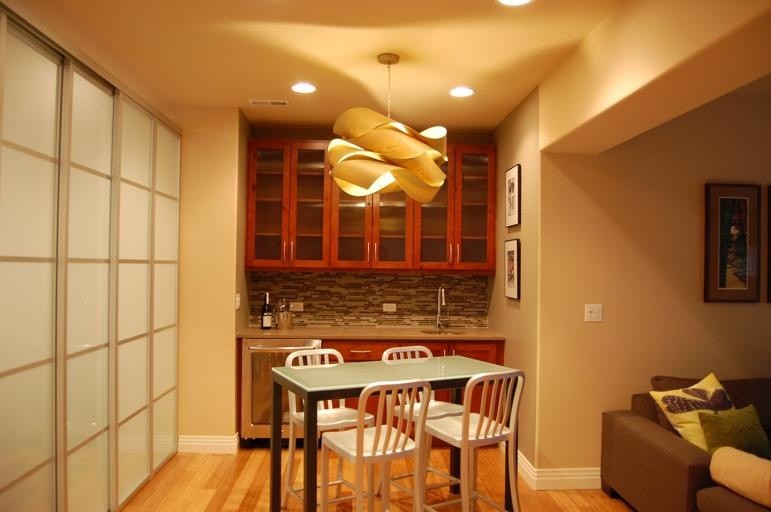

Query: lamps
(327, 52), (450, 205)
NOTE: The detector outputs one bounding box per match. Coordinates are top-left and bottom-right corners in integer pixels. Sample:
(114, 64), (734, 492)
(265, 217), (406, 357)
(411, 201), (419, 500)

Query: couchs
(600, 375), (771, 512)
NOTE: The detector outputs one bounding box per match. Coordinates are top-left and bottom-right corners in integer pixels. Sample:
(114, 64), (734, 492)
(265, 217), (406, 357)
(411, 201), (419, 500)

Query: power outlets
(584, 304), (602, 322)
(382, 303), (396, 312)
(235, 293), (241, 311)
(289, 302), (304, 313)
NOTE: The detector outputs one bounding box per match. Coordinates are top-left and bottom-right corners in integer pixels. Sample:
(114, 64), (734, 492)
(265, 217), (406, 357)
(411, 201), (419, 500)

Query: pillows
(649, 372), (738, 451)
(697, 404), (771, 459)
(710, 445), (771, 509)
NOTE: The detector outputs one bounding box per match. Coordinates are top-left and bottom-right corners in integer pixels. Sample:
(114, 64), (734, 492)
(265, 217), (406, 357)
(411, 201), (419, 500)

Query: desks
(270, 355), (522, 512)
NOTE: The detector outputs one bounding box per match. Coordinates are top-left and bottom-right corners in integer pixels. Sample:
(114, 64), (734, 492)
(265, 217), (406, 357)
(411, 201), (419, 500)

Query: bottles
(260, 292), (272, 330)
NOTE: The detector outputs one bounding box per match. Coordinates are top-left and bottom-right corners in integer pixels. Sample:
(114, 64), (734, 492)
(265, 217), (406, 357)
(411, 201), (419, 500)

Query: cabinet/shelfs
(321, 340), (401, 452)
(413, 142), (497, 277)
(244, 139), (330, 273)
(330, 142), (412, 276)
(400, 340), (506, 450)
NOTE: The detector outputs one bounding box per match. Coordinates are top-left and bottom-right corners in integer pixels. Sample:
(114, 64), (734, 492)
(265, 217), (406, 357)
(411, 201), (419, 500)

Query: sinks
(421, 328), (467, 336)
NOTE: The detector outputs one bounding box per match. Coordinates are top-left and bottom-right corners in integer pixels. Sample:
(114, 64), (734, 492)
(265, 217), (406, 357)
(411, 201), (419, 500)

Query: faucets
(436, 283), (446, 327)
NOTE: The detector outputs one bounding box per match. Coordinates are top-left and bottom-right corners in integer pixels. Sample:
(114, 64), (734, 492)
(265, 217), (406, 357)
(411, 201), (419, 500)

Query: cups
(276, 311), (288, 330)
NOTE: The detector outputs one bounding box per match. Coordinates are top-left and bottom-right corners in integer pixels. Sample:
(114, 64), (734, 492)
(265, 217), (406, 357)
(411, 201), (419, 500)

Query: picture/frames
(505, 164), (521, 228)
(767, 185), (771, 304)
(504, 238), (520, 301)
(704, 182), (761, 303)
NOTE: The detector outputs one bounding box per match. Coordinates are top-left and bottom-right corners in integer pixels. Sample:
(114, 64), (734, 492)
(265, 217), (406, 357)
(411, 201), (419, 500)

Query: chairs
(321, 379), (432, 512)
(375, 345), (462, 503)
(280, 348), (376, 512)
(422, 370), (525, 512)
(695, 444), (771, 512)
